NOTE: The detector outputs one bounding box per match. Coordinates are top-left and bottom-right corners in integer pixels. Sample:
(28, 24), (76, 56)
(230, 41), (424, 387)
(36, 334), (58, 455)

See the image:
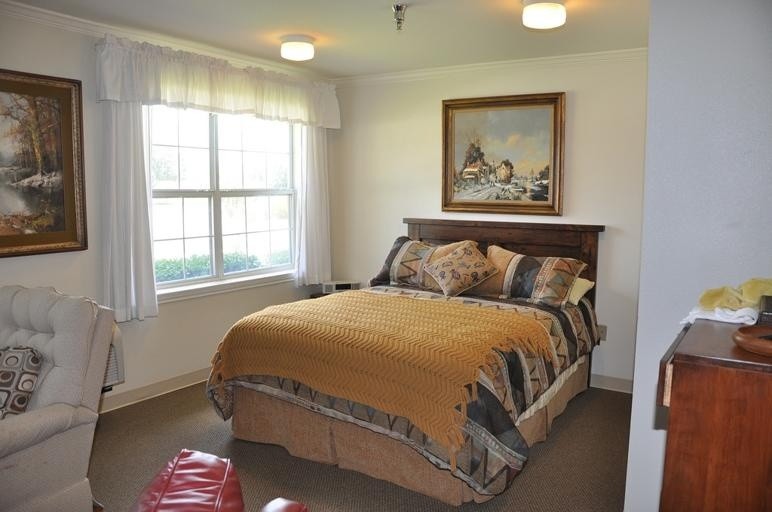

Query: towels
(677, 276), (772, 327)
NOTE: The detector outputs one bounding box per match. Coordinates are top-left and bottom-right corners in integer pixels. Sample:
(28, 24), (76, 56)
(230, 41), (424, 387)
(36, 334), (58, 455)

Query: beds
(231, 218), (605, 506)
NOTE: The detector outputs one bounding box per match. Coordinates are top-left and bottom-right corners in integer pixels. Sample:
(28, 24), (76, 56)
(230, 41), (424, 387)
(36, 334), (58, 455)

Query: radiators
(101, 322), (126, 388)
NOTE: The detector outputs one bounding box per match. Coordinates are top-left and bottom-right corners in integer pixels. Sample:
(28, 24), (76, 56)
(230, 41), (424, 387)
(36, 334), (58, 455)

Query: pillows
(0, 346), (42, 421)
(366, 235), (596, 312)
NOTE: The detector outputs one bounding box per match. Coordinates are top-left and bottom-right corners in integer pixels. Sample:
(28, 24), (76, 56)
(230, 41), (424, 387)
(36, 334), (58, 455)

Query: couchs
(0, 286), (115, 512)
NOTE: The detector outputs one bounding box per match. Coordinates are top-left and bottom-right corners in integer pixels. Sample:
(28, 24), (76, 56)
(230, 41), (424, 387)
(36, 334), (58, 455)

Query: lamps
(280, 35), (316, 61)
(519, 0), (566, 29)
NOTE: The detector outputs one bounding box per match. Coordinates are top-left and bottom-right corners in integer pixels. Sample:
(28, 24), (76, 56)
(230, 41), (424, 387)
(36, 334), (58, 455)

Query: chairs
(126, 447), (310, 512)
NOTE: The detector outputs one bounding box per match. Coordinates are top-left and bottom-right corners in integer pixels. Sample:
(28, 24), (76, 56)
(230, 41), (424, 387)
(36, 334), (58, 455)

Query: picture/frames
(440, 92), (566, 216)
(0, 69), (89, 258)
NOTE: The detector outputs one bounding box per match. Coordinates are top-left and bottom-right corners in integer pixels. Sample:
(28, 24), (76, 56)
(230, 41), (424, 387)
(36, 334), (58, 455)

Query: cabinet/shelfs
(653, 313), (772, 512)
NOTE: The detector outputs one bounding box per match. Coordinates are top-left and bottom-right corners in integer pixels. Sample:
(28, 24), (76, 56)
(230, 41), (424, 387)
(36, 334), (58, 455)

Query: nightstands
(308, 291), (331, 300)
